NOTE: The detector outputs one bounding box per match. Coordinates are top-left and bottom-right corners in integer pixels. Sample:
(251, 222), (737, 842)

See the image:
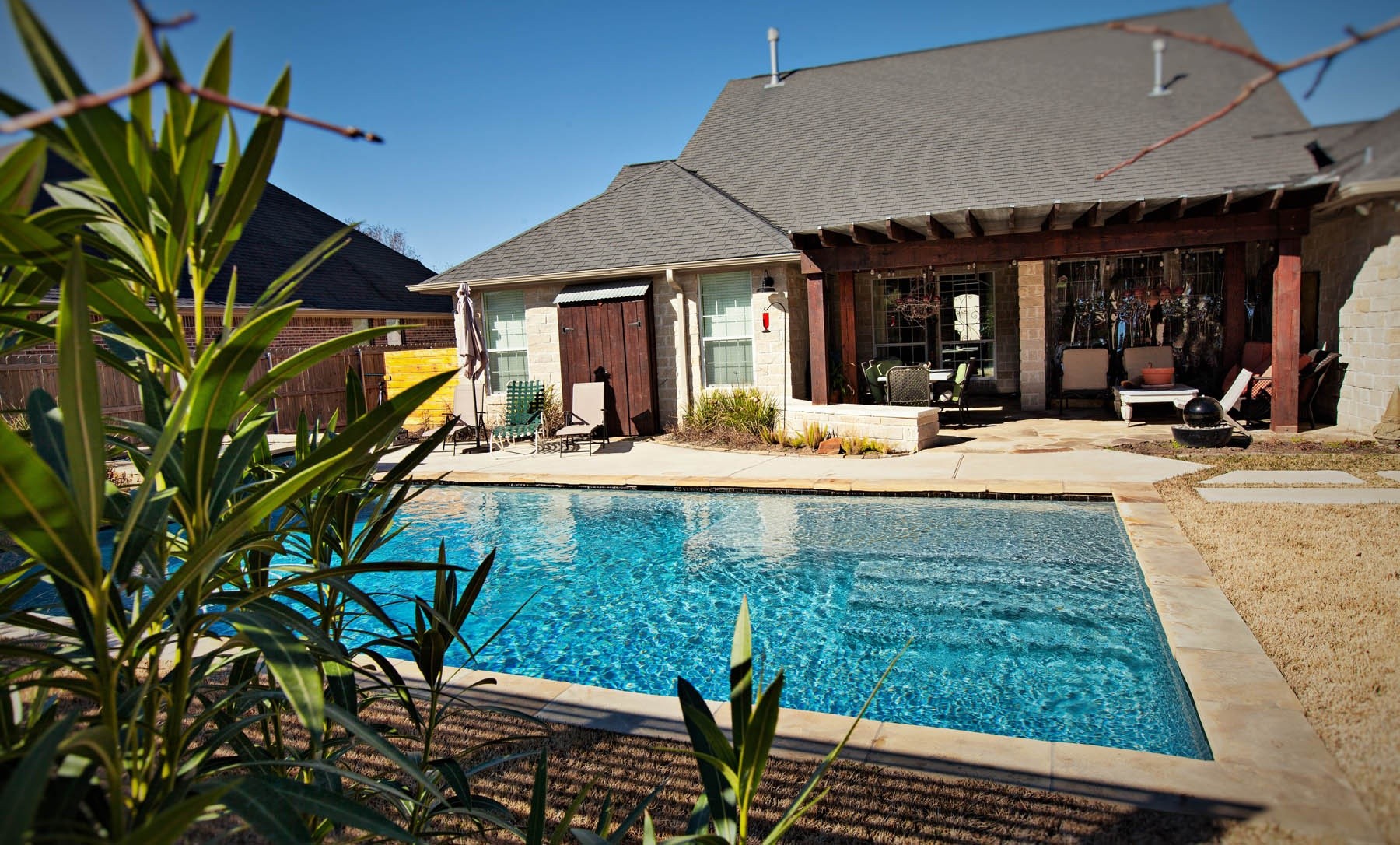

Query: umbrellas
(456, 282), (488, 447)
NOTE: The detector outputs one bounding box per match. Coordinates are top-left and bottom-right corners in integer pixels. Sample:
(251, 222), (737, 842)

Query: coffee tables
(1111, 384), (1199, 423)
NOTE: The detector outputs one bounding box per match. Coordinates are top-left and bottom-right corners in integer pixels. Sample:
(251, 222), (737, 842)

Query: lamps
(762, 301), (786, 433)
(757, 269), (775, 293)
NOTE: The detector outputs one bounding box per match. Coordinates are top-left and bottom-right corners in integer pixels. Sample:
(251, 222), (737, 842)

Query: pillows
(1222, 352), (1318, 394)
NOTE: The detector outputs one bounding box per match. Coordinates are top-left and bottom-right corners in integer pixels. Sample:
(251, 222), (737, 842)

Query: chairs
(490, 379), (548, 456)
(556, 382), (609, 458)
(1174, 368), (1254, 436)
(422, 384), (489, 456)
(1058, 348), (1109, 415)
(1120, 344), (1173, 383)
(861, 358), (973, 427)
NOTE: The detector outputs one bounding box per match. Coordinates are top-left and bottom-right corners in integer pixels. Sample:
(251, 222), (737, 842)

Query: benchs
(1222, 342), (1342, 429)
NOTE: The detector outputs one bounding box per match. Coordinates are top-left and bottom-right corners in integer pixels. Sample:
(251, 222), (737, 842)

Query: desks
(878, 367), (955, 382)
